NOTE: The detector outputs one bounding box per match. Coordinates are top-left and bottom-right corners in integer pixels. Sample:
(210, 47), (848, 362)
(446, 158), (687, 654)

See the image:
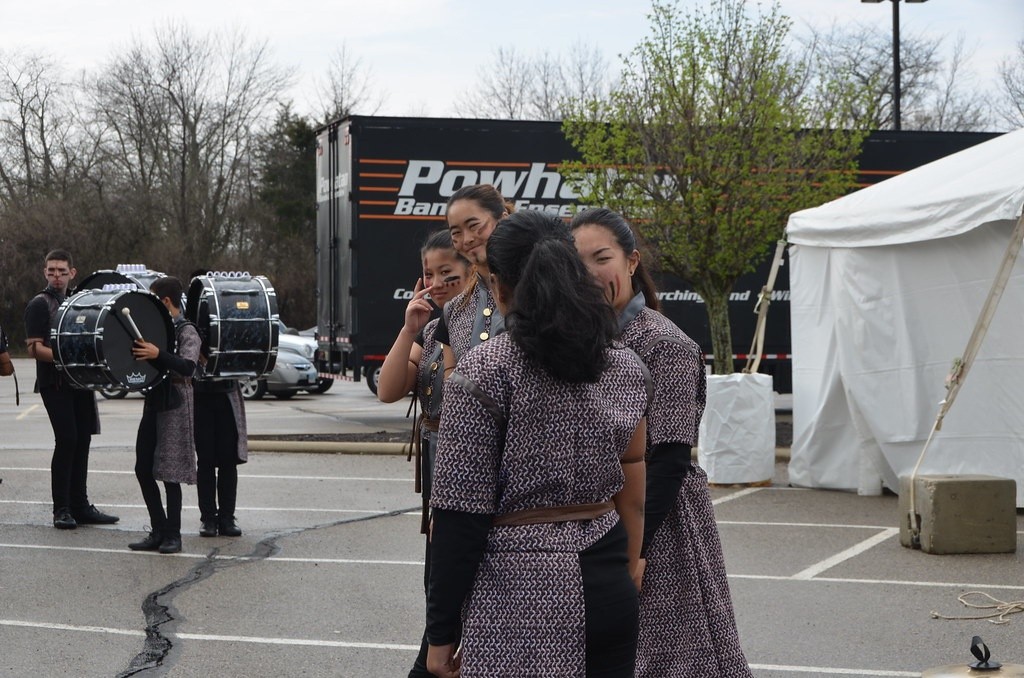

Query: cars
(237, 317), (336, 402)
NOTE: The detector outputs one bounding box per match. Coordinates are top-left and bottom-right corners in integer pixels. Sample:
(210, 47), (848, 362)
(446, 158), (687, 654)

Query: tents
(785, 127), (1023, 511)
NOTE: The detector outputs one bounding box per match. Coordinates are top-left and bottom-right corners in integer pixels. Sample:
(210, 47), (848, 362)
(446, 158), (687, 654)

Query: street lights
(860, 0), (930, 129)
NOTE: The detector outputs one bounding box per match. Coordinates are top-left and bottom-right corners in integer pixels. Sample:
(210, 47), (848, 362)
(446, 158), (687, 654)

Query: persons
(0, 325), (15, 378)
(402, 184), (517, 678)
(23, 249), (120, 529)
(186, 267), (249, 537)
(426, 209), (649, 678)
(567, 209), (754, 678)
(127, 276), (202, 555)
(376, 229), (475, 598)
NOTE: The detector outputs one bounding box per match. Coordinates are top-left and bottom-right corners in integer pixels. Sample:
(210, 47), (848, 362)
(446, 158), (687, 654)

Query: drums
(186, 271), (282, 383)
(49, 282), (177, 394)
(68, 263), (189, 320)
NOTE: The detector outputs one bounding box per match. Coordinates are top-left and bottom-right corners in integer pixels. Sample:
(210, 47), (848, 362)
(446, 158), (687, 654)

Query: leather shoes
(157, 533), (182, 553)
(126, 528), (162, 550)
(78, 504), (121, 524)
(215, 516), (241, 537)
(199, 516), (217, 537)
(53, 506), (77, 529)
